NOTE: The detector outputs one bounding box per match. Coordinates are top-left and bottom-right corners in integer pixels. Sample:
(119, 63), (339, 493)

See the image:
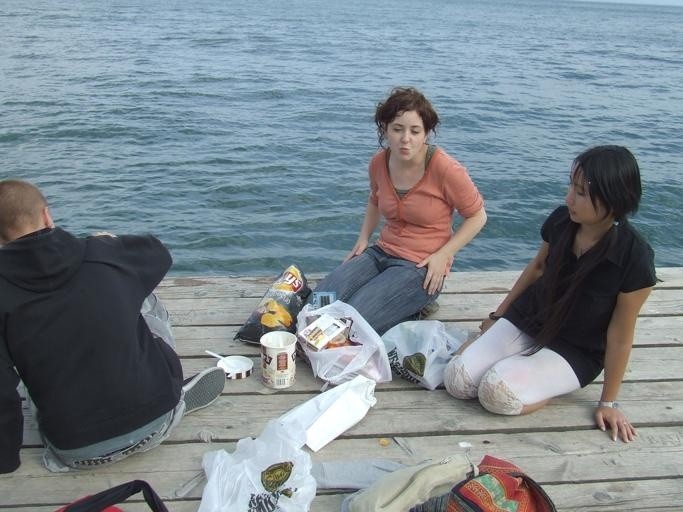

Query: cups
(259, 330), (298, 390)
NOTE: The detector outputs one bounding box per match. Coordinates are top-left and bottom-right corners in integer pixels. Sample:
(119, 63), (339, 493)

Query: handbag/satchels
(343, 456), (479, 511)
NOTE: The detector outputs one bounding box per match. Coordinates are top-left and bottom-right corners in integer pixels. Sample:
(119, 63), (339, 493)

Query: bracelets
(488, 312), (502, 322)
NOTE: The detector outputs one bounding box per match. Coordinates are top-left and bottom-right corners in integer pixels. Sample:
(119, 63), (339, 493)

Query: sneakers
(182, 367), (226, 415)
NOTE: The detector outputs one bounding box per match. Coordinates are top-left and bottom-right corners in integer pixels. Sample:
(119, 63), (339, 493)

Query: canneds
(327, 334), (350, 349)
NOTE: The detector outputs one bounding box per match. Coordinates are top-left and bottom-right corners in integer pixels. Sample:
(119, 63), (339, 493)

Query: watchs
(599, 401), (621, 409)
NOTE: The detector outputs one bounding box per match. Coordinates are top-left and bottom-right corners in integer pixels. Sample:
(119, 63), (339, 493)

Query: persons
(0, 179), (226, 475)
(442, 143), (657, 443)
(302, 86), (489, 339)
